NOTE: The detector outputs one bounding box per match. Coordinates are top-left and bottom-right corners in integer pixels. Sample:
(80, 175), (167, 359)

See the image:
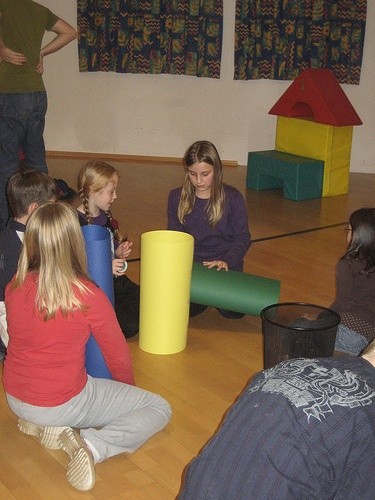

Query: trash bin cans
(260, 301), (343, 370)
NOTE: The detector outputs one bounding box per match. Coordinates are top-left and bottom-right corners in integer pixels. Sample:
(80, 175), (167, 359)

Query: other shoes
(18, 417), (73, 450)
(57, 425), (98, 491)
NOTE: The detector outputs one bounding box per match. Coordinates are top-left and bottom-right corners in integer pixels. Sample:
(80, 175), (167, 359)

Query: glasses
(344, 224), (352, 232)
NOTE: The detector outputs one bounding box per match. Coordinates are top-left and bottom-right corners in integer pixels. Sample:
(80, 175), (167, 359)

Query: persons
(166, 141), (252, 320)
(0, 159), (173, 490)
(0, 0), (79, 230)
(300, 207), (375, 358)
(175, 338), (375, 500)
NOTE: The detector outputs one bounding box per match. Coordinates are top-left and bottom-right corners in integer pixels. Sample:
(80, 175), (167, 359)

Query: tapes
(118, 261), (128, 273)
